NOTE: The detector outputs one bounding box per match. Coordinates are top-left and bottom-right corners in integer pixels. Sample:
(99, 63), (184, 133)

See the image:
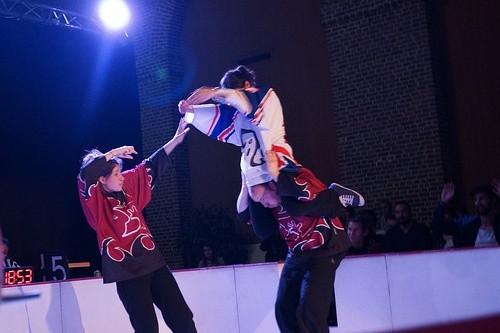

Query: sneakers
(328, 182), (365, 209)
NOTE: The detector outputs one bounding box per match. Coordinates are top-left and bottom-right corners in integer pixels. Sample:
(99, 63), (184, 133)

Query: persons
(0, 237), (20, 268)
(198, 243), (225, 267)
(422, 209), (461, 250)
(384, 201), (430, 252)
(339, 204), (354, 226)
(384, 215), (397, 232)
(236, 168), (352, 333)
(431, 179), (500, 246)
(345, 216), (383, 255)
(356, 209), (384, 248)
(378, 199), (393, 229)
(77, 117), (198, 333)
(179, 64), (366, 262)
